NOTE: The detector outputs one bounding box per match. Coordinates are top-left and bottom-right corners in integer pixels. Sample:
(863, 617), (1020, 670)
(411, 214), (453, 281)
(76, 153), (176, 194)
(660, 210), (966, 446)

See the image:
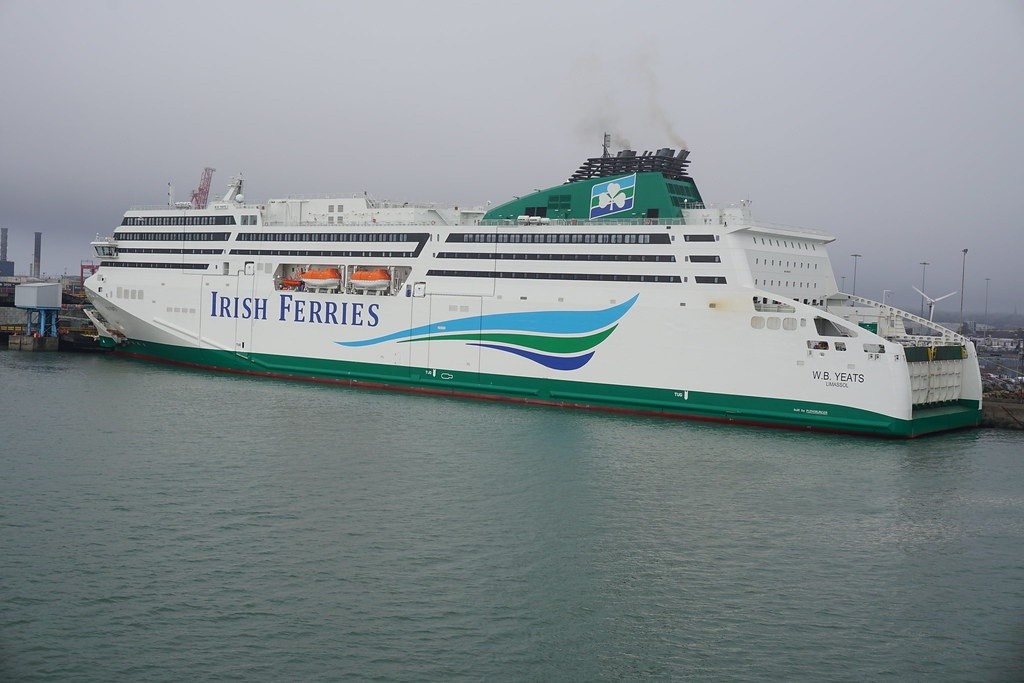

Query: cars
(976, 344), (1024, 402)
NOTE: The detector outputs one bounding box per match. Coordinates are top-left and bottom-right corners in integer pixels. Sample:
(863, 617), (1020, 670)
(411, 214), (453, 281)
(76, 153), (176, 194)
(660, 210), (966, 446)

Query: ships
(83, 132), (987, 439)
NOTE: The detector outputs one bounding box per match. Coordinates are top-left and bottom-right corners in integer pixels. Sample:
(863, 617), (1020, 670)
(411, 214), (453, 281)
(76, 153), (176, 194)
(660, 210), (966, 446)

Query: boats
(350, 269), (391, 290)
(300, 268), (342, 290)
